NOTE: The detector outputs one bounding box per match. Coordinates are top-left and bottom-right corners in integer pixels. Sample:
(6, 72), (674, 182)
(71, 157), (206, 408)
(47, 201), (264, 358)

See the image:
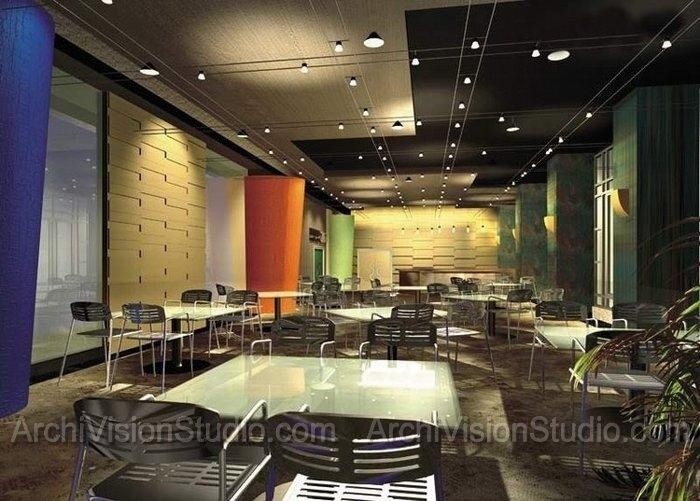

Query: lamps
(300, 63), (309, 74)
(334, 30), (404, 135)
(236, 130), (250, 140)
(138, 60), (160, 76)
(197, 70), (206, 82)
